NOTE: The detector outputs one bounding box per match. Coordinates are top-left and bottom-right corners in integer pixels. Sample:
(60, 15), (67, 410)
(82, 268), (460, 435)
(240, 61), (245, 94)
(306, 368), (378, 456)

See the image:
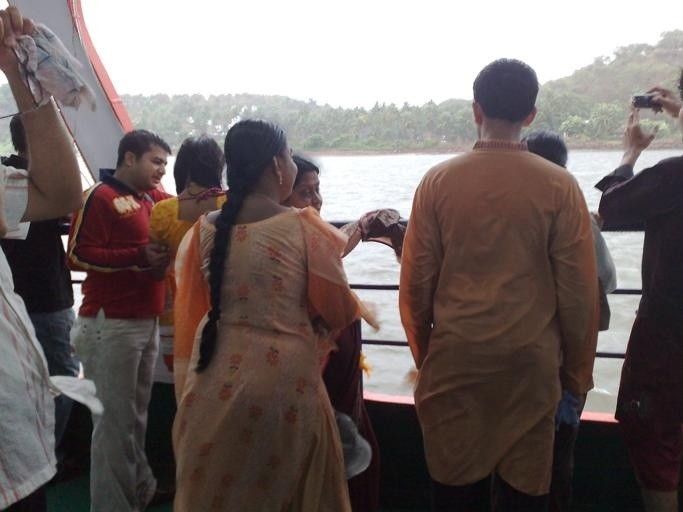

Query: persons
(0, 6), (83, 512)
(171, 119), (363, 510)
(524, 129), (618, 510)
(64, 130), (176, 511)
(593, 64), (683, 511)
(399, 57), (600, 511)
(152, 137), (228, 375)
(279, 154), (398, 510)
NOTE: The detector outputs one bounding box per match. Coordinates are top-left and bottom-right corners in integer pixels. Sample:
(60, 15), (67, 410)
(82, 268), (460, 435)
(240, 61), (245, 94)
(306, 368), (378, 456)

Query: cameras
(632, 95), (657, 107)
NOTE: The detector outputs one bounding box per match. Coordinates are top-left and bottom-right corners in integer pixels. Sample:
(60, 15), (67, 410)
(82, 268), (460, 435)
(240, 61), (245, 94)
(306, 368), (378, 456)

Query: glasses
(0, 44), (44, 119)
